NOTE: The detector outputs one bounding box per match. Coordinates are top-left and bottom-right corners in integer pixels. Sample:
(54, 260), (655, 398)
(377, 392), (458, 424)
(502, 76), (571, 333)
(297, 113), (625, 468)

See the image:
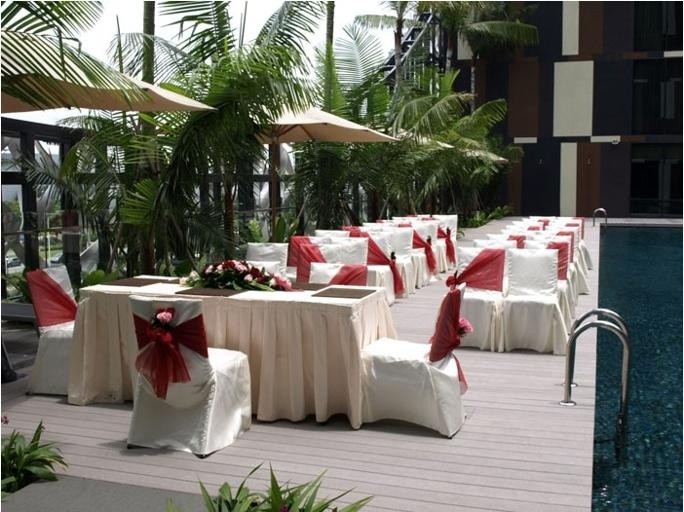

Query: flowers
(179, 255), (296, 292)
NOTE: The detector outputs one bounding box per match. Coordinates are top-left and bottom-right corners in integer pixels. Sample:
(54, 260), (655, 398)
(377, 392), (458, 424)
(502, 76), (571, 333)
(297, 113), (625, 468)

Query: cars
(7, 255), (22, 267)
(50, 252), (64, 264)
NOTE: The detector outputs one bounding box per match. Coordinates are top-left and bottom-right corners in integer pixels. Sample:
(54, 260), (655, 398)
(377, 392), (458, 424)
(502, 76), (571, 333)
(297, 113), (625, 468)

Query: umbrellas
(235, 99), (401, 243)
(1, 31), (227, 121)
(360, 118), (512, 171)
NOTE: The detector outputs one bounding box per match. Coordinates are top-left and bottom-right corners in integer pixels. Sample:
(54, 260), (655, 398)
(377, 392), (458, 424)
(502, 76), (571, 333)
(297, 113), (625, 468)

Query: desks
(64, 274), (399, 434)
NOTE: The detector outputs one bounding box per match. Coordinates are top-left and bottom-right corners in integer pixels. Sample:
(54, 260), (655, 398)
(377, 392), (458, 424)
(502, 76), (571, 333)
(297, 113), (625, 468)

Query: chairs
(245, 214), (459, 306)
(26, 264), (77, 398)
(127, 293), (253, 457)
(453, 214), (596, 358)
(360, 282), (473, 440)
